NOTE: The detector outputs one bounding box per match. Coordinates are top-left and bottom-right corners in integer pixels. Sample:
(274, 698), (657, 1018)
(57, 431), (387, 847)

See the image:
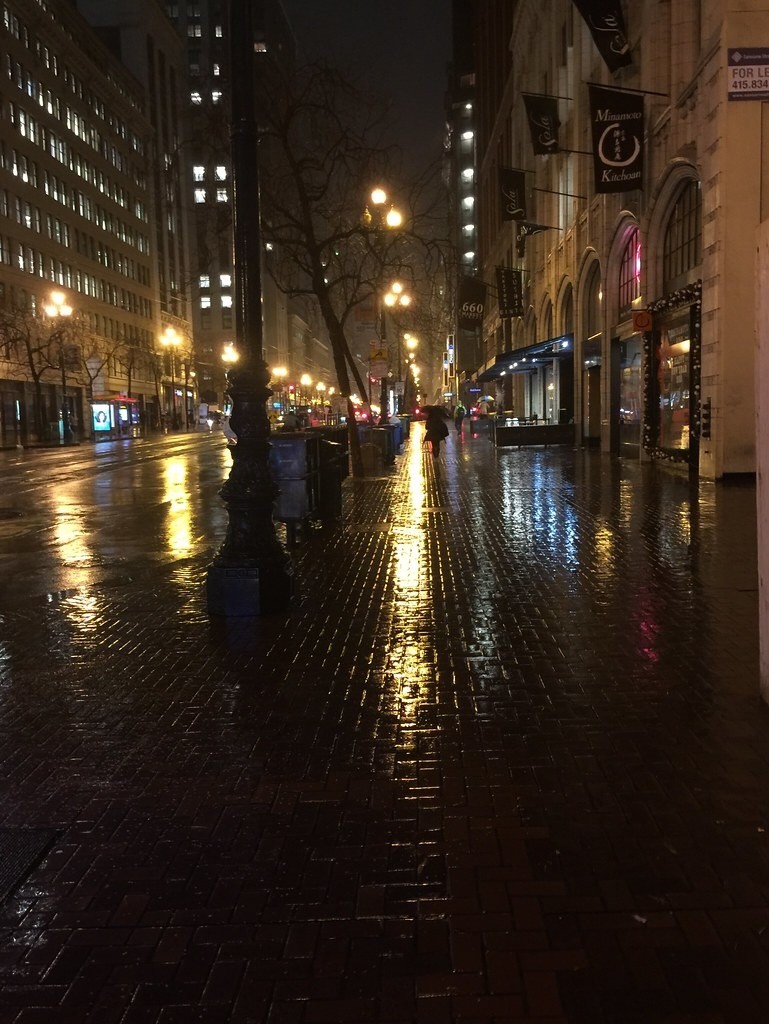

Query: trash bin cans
(319, 438), (343, 519)
(396, 413), (413, 437)
(373, 423), (401, 456)
(361, 427), (393, 468)
(270, 430), (321, 550)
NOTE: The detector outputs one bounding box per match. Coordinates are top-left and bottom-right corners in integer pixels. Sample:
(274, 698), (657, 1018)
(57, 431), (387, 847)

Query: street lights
(384, 281), (411, 415)
(300, 374), (313, 406)
(45, 290), (73, 446)
(162, 325), (182, 429)
(273, 368), (288, 416)
(315, 382), (327, 421)
(358, 185), (404, 464)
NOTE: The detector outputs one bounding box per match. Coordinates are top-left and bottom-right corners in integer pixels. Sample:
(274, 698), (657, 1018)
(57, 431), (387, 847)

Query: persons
(69, 412), (79, 443)
(454, 400), (466, 435)
(286, 412), (300, 429)
(478, 400), (490, 420)
(208, 410), (222, 424)
(189, 410), (193, 424)
(425, 415), (449, 458)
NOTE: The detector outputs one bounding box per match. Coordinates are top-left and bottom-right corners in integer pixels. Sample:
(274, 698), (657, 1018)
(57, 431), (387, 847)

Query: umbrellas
(421, 405), (449, 414)
(477, 395), (495, 402)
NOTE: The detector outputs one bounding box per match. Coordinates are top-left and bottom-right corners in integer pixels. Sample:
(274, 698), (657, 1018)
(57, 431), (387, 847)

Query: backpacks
(455, 405), (464, 416)
(440, 421), (449, 437)
